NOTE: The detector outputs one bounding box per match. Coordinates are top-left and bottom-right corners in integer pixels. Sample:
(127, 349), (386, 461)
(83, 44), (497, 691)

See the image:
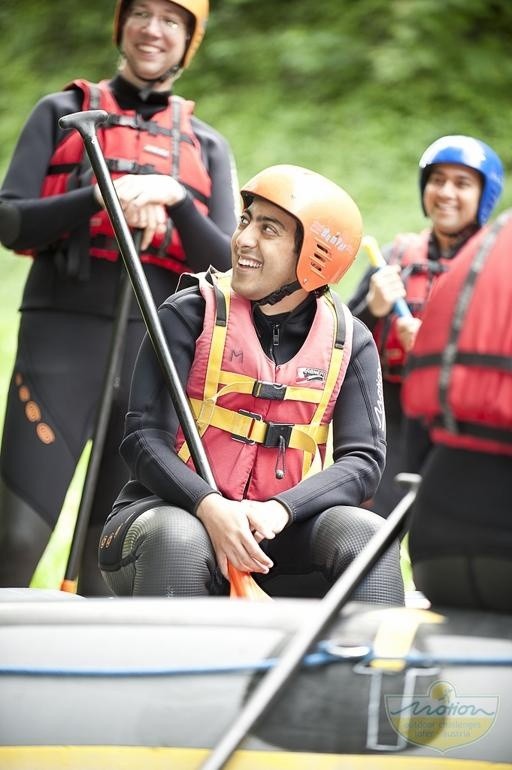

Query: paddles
(59, 110), (272, 601)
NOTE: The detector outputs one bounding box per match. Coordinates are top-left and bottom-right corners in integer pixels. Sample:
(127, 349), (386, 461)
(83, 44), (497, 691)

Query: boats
(0, 581), (512, 769)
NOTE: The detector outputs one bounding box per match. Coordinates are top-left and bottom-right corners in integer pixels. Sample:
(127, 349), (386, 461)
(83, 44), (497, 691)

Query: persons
(0, 0), (244, 597)
(399, 207), (512, 618)
(348, 135), (504, 532)
(98, 161), (405, 608)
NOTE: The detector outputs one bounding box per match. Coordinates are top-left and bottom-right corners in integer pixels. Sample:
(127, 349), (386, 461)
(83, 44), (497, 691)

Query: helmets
(113, 0), (210, 70)
(239, 164), (364, 293)
(418, 134), (504, 225)
